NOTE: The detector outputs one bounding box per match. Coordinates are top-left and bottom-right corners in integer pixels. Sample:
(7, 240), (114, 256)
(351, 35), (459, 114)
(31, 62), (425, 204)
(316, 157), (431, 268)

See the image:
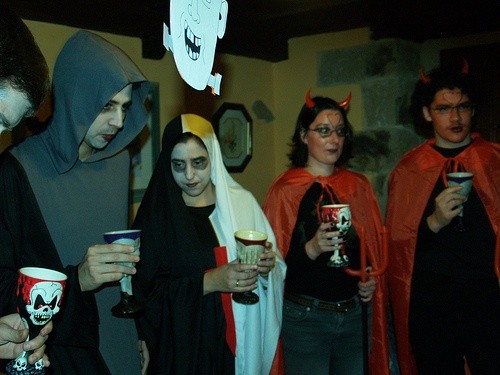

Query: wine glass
(231, 231), (268, 305)
(447, 172), (474, 233)
(102, 230), (146, 316)
(4, 266), (68, 375)
(321, 204), (352, 268)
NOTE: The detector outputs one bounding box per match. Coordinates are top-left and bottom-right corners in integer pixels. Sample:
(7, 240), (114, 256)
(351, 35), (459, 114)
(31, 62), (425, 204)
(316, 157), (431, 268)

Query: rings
(236, 280), (239, 286)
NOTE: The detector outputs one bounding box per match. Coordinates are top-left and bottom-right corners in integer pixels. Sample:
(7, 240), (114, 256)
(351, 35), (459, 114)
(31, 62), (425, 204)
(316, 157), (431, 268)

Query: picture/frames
(124, 81), (161, 204)
(215, 101), (253, 174)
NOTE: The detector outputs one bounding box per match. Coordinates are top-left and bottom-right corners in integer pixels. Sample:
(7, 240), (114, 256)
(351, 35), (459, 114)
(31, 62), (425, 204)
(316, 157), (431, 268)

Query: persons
(386, 59), (500, 375)
(131, 114), (288, 375)
(14, 33), (149, 375)
(1, 0), (67, 375)
(262, 89), (390, 375)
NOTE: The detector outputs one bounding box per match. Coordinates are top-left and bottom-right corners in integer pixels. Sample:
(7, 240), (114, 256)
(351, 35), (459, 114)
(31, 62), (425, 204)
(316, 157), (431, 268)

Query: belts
(283, 290), (360, 313)
